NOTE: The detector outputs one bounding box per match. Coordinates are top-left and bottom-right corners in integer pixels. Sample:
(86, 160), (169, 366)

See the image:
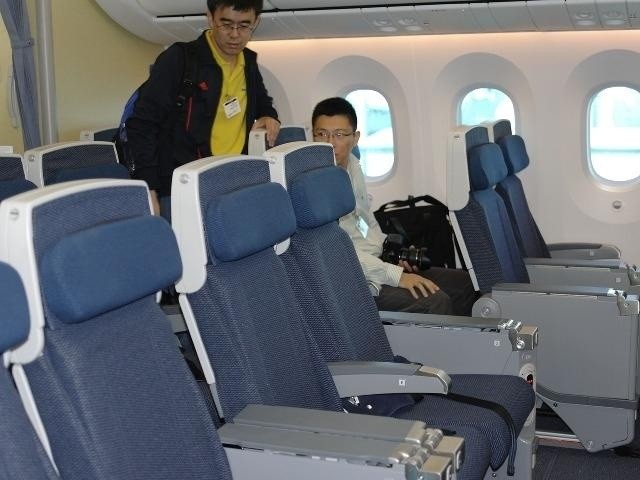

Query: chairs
(167, 152), (494, 480)
(0, 180), (237, 480)
(449, 120), (638, 453)
(262, 138), (536, 473)
(1, 120), (360, 337)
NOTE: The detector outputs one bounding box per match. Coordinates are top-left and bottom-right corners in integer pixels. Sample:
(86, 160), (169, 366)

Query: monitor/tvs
(381, 233), (431, 269)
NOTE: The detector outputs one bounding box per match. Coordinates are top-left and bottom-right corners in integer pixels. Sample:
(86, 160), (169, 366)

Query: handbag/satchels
(373, 195), (456, 269)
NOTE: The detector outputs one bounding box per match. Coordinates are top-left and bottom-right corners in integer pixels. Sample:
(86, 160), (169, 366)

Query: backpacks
(113, 42), (197, 196)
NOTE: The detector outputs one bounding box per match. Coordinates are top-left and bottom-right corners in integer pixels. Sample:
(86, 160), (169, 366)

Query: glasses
(214, 19), (255, 31)
(313, 130), (355, 139)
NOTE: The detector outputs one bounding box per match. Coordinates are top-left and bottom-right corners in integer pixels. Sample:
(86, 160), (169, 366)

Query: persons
(120, 0), (281, 217)
(311, 97), (476, 315)
